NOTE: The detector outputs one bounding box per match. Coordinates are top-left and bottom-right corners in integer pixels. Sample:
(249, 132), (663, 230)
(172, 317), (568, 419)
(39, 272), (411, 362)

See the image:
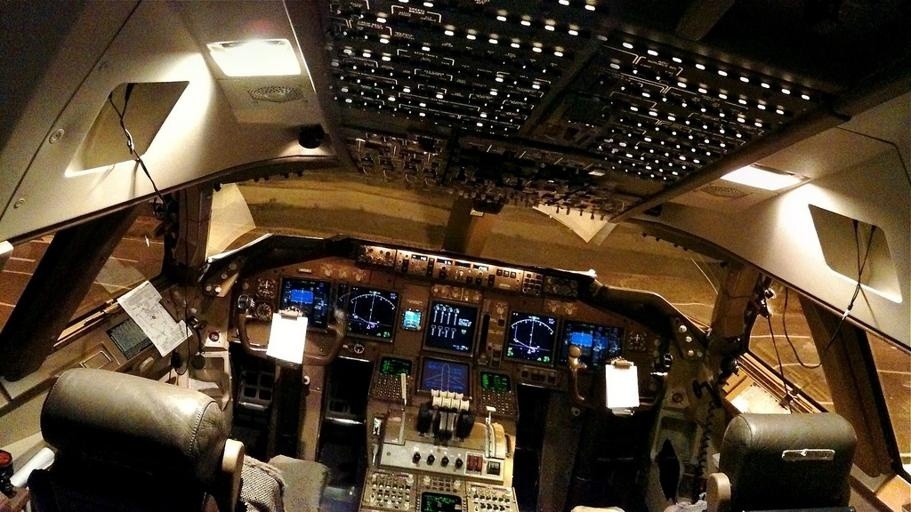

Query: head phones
(171, 321), (206, 371)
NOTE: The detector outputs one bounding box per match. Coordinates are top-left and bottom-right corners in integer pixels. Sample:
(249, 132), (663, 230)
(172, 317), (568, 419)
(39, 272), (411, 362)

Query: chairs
(705, 414), (858, 510)
(29, 371), (244, 512)
